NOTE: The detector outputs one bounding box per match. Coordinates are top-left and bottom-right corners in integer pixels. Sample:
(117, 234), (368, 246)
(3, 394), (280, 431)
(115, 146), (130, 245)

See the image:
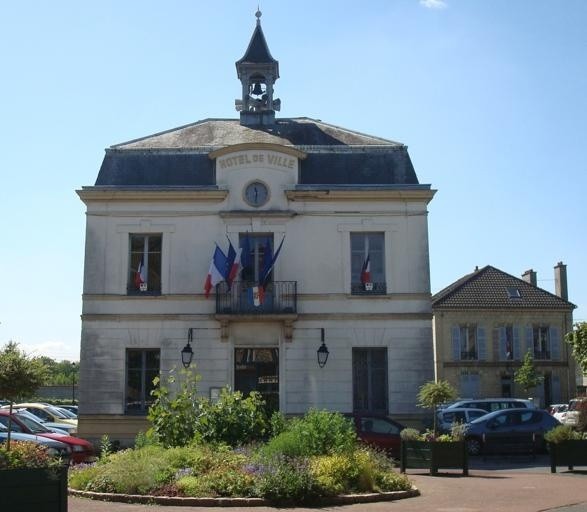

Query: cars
(424, 397), (586, 455)
(0, 400), (97, 467)
(322, 414), (408, 466)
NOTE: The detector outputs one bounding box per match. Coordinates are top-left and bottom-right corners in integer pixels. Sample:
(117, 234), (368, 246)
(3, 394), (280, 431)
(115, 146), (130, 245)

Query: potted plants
(543, 424), (586, 473)
(399, 379), (471, 477)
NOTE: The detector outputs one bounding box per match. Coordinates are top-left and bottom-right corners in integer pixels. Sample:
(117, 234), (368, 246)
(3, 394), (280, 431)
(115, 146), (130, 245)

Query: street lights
(72, 362), (75, 405)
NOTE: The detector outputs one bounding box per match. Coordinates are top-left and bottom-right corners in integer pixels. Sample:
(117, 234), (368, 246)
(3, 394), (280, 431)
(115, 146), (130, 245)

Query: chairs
(363, 420), (372, 430)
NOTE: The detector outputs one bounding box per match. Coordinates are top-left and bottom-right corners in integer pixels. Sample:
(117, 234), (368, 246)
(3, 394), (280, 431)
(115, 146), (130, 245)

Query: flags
(135, 255), (144, 289)
(360, 253), (371, 286)
(205, 231), (285, 307)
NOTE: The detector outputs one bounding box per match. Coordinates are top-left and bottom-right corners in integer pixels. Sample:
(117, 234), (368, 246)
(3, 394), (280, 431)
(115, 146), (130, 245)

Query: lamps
(180, 327), (193, 369)
(316, 327), (329, 367)
